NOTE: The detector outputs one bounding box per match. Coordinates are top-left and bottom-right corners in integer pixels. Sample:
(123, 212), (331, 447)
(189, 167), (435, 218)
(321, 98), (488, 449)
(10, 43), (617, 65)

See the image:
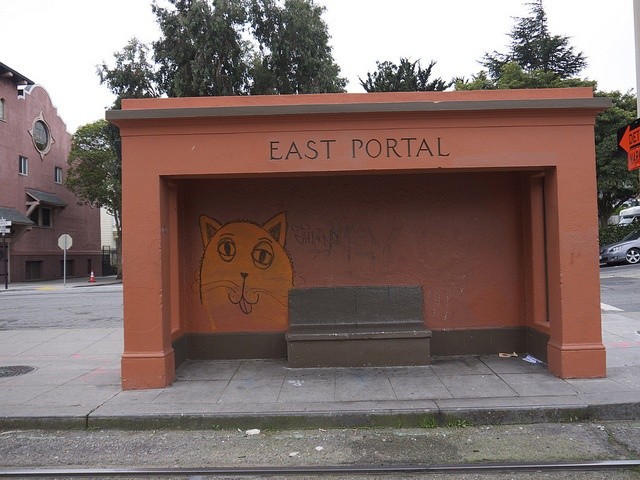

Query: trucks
(616, 205), (640, 226)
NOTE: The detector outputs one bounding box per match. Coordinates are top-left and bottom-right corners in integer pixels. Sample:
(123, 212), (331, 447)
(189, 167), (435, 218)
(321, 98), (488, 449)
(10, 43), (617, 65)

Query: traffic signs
(617, 117), (640, 172)
(1, 228), (10, 233)
(0, 220), (12, 226)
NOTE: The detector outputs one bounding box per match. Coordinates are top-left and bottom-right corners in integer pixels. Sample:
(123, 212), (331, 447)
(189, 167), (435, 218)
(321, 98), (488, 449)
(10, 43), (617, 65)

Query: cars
(599, 229), (640, 264)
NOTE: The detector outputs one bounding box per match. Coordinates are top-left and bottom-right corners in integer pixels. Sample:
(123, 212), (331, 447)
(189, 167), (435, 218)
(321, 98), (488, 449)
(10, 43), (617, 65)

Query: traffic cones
(89, 269), (97, 282)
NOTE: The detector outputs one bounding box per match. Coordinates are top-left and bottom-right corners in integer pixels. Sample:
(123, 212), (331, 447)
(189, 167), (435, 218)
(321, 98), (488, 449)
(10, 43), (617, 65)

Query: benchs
(284, 285), (433, 368)
